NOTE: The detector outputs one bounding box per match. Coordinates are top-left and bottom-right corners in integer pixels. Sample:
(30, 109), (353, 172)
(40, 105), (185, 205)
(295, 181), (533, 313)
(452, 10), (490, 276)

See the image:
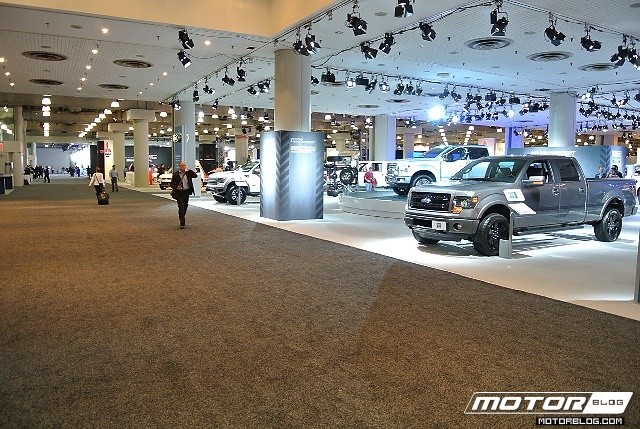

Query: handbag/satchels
(170, 190), (178, 199)
(99, 183), (103, 189)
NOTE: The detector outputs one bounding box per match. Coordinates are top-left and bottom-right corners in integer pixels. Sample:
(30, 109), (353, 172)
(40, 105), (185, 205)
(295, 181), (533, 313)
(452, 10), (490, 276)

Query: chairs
(526, 167), (546, 178)
(495, 168), (511, 179)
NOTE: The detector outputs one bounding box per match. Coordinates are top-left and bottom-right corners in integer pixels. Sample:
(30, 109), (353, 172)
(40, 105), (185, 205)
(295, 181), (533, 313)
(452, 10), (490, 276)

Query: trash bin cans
(5, 176), (12, 189)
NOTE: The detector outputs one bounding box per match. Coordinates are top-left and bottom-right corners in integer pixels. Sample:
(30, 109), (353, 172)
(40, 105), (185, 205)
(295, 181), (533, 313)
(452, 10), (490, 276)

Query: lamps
(419, 21), (437, 42)
(192, 89), (200, 102)
(304, 34), (322, 54)
(523, 129), (532, 138)
(544, 25), (567, 46)
(611, 45), (629, 67)
(394, 0), (417, 19)
(168, 99), (182, 110)
(451, 84), (462, 102)
(459, 91), (505, 125)
(577, 84), (640, 133)
(503, 108), (515, 119)
(221, 75), (236, 87)
(247, 86), (259, 96)
(403, 117), (418, 129)
(438, 83), (450, 99)
(582, 34), (603, 53)
(311, 68), (424, 97)
(203, 85), (215, 96)
(627, 48), (640, 69)
(293, 40), (309, 56)
(177, 51), (192, 69)
(236, 66), (247, 82)
(359, 41), (378, 60)
(379, 32), (397, 54)
(347, 11), (369, 35)
(240, 107), (270, 134)
(509, 94), (520, 105)
(212, 101), (219, 110)
(542, 129), (549, 140)
(519, 97), (549, 116)
(178, 30), (195, 49)
(330, 116), (375, 139)
(490, 8), (510, 37)
(512, 130), (519, 137)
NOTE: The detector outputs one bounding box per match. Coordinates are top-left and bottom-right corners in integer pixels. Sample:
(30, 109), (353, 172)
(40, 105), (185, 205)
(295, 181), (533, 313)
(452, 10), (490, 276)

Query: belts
(112, 177), (116, 178)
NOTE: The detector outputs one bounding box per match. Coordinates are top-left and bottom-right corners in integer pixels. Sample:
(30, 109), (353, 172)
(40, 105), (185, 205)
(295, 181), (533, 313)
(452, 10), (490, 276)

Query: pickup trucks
(404, 156), (637, 256)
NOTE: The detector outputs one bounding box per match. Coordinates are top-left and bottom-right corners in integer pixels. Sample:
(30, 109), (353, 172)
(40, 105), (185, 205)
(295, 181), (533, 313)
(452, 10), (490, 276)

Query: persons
(109, 164), (120, 193)
(67, 166), (80, 177)
(88, 167), (106, 199)
(170, 162), (198, 230)
(148, 164), (169, 183)
(25, 163), (55, 184)
(608, 165), (622, 178)
(364, 167), (374, 192)
(595, 164), (607, 179)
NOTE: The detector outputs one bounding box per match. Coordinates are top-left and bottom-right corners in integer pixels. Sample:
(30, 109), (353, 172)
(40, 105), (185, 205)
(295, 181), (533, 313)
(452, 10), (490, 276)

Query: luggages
(97, 191), (109, 204)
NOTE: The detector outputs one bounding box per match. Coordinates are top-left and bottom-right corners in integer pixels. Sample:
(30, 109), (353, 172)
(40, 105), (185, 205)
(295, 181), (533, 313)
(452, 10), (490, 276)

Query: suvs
(158, 159), (203, 189)
(206, 158), (327, 204)
(386, 144), (488, 194)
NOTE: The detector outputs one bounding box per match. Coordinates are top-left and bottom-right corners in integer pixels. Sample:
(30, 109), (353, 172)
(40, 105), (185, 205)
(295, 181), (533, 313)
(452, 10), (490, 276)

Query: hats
(112, 165), (115, 167)
(96, 168), (101, 172)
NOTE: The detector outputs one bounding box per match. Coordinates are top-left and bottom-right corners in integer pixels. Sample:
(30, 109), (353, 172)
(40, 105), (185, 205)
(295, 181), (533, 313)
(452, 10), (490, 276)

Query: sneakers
(180, 225), (185, 229)
